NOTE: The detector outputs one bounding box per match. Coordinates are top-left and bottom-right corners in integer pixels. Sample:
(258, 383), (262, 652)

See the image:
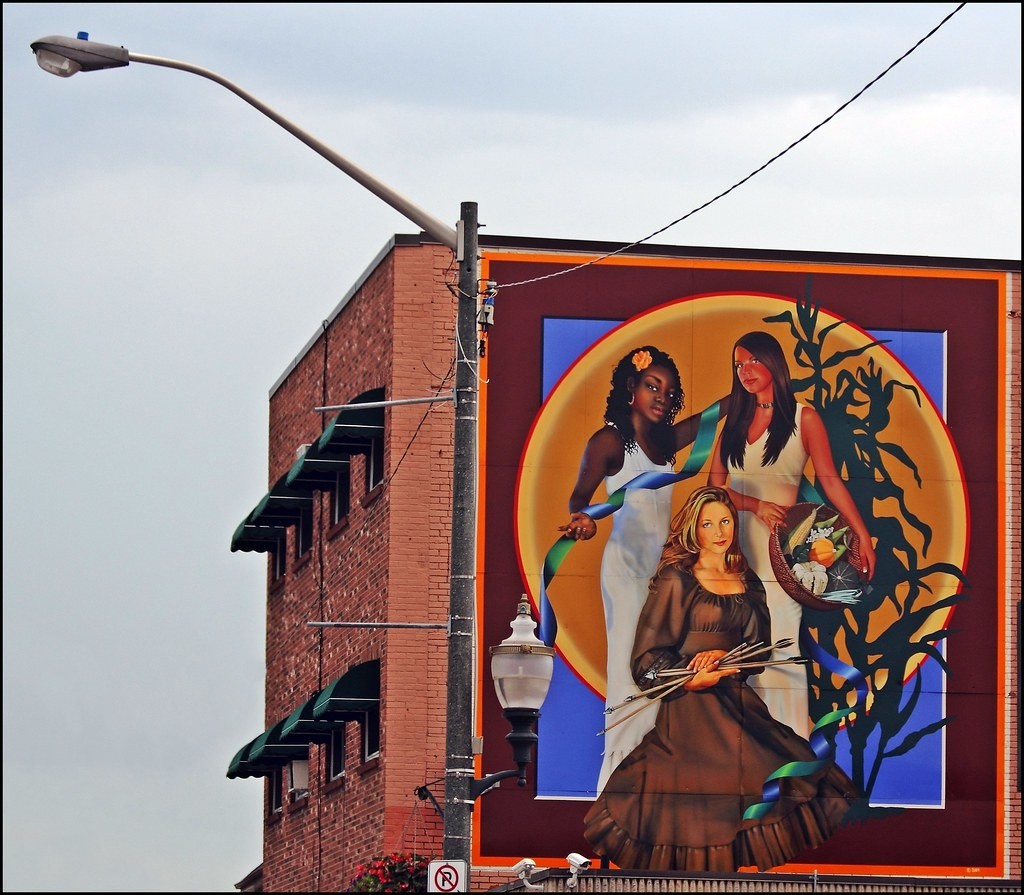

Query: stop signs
(428, 860), (466, 893)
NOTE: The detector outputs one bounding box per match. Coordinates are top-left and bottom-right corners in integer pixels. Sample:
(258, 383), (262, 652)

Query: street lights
(30, 34), (557, 893)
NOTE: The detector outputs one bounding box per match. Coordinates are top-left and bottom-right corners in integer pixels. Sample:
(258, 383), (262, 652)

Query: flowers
(346, 851), (433, 892)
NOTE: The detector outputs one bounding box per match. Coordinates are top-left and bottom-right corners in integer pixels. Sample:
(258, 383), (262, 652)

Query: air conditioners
(285, 760), (309, 793)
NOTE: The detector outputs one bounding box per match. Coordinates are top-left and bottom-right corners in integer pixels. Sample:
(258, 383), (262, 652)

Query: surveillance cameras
(512, 858), (536, 874)
(566, 853), (592, 870)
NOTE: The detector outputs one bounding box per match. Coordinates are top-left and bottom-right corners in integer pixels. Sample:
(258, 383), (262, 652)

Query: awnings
(227, 658), (377, 780)
(231, 386), (382, 554)
